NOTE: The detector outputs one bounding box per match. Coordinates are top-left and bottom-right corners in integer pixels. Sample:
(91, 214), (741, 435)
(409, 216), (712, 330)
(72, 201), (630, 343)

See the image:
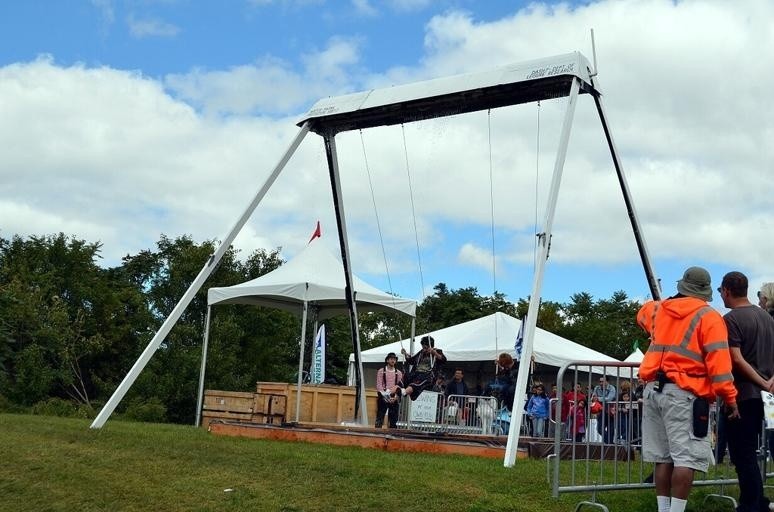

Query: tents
(348, 312), (639, 398)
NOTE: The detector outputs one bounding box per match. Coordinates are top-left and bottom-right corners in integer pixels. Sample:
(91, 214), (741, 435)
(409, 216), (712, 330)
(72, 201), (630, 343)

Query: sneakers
(395, 386), (403, 404)
(378, 391), (393, 404)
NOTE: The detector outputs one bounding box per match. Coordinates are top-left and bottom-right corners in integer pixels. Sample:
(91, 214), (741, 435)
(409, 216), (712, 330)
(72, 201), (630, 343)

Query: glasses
(717, 287), (721, 292)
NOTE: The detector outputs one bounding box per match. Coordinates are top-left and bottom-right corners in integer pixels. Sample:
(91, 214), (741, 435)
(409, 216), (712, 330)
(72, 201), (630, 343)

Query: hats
(386, 353), (398, 361)
(678, 268), (712, 301)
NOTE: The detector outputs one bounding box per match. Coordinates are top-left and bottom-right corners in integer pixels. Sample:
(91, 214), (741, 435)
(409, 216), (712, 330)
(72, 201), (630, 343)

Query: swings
(487, 101), (546, 405)
(359, 122), (444, 393)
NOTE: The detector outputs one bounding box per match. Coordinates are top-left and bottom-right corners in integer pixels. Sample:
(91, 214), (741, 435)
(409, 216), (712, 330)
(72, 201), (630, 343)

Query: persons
(717, 271), (773, 512)
(637, 266), (741, 512)
(529, 374), (647, 445)
(377, 335), (447, 404)
(375, 352), (403, 429)
(433, 354), (520, 435)
